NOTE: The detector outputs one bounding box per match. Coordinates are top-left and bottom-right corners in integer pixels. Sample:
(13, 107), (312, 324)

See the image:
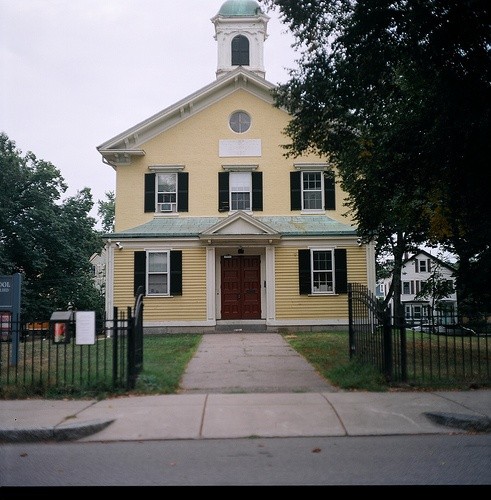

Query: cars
(410, 323), (476, 337)
